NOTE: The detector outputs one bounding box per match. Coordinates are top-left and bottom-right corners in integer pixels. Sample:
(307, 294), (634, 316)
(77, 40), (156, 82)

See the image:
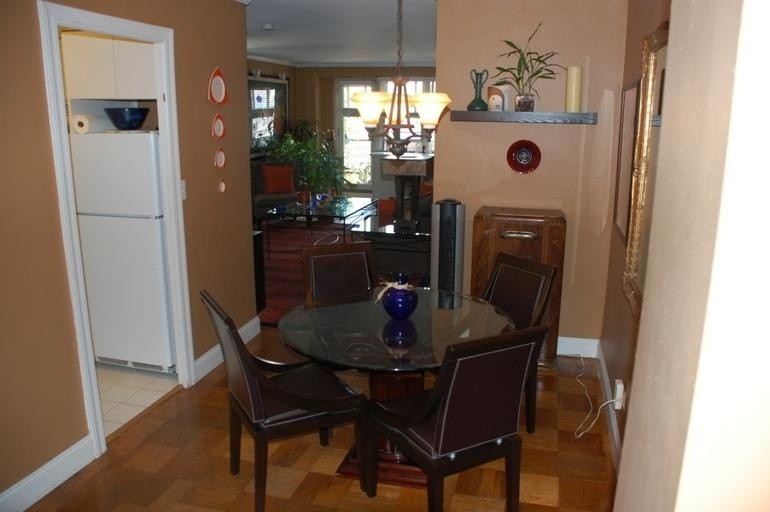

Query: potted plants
(490, 22), (568, 113)
(263, 120), (353, 204)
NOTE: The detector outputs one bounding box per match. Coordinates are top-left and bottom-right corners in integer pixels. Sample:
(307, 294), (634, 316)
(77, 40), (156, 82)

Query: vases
(467, 69), (489, 111)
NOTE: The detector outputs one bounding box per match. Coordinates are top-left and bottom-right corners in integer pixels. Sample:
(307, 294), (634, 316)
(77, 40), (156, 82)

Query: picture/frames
(612, 17), (669, 316)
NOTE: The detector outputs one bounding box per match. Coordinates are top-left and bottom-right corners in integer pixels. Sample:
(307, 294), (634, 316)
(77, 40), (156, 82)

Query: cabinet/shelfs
(470, 205), (567, 369)
(61, 34), (158, 101)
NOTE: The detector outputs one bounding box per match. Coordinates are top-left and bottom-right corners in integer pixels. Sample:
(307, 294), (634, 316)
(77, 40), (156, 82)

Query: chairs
(434, 251), (556, 436)
(301, 247), (376, 438)
(199, 287), (367, 511)
(364, 324), (550, 511)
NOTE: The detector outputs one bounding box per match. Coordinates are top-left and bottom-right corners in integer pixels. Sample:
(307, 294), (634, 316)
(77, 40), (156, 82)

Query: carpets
(254, 220), (432, 328)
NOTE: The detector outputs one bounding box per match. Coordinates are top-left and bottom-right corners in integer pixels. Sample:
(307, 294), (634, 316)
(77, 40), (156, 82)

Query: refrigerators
(69, 132), (177, 375)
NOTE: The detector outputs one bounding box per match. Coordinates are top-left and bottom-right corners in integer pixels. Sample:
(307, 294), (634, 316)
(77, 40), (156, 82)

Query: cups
(297, 192), (310, 203)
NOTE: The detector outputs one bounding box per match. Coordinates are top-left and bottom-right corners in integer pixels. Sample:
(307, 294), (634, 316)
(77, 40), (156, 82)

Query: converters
(613, 379), (624, 410)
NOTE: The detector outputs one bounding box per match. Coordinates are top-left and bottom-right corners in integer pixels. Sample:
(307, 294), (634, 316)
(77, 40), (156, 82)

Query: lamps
(350, 0), (453, 162)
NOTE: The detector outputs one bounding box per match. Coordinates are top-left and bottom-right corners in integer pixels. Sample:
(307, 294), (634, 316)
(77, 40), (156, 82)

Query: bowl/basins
(105, 107), (149, 128)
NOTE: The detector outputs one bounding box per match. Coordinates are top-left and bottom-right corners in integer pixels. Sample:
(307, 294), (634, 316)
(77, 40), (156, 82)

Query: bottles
(384, 274), (418, 320)
